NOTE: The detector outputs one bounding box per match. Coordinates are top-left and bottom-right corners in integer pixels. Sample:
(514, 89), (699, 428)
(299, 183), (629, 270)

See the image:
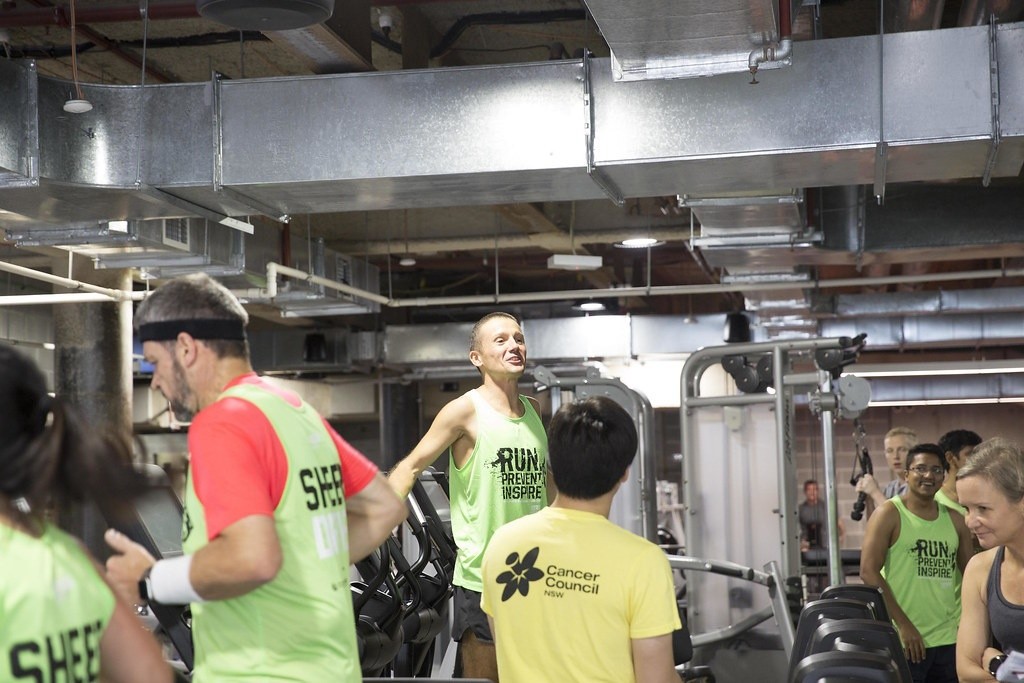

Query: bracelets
(148, 553), (204, 608)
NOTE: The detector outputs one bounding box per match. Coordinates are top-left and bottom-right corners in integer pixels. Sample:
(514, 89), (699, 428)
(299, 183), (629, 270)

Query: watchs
(988, 654), (1010, 676)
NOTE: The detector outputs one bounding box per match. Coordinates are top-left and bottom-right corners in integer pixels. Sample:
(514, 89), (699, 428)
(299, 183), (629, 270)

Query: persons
(477, 393), (689, 683)
(953, 436), (1024, 683)
(100, 273), (413, 683)
(1, 337), (175, 683)
(856, 441), (978, 683)
(391, 312), (567, 683)
(796, 479), (847, 593)
(853, 425), (918, 528)
(936, 429), (985, 556)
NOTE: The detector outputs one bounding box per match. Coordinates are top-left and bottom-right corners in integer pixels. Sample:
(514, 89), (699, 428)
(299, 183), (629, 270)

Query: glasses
(909, 466), (943, 474)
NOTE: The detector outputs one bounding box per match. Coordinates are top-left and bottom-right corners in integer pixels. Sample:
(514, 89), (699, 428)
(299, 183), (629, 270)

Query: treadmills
(89, 461), (464, 682)
